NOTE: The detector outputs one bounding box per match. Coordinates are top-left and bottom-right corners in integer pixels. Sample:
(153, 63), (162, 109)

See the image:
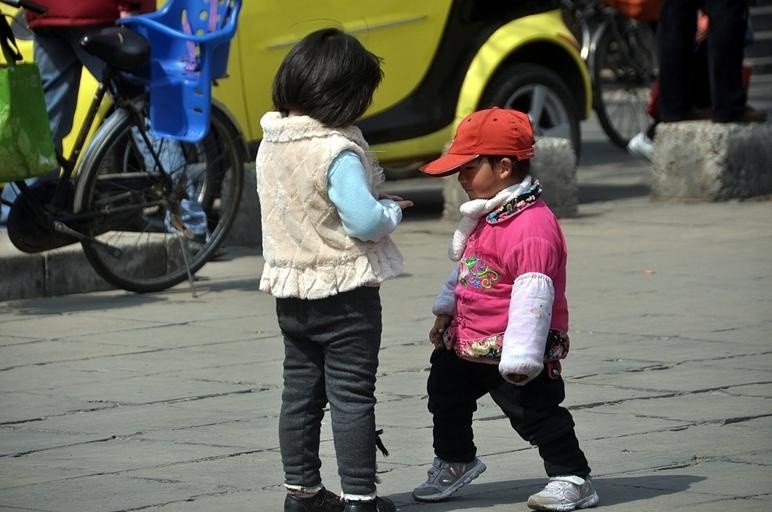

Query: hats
(418, 106), (535, 177)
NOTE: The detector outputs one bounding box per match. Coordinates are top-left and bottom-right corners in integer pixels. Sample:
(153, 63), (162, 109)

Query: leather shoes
(342, 496), (398, 512)
(283, 487), (344, 511)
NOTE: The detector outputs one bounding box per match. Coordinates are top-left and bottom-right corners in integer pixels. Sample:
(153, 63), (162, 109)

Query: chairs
(115, 0), (244, 145)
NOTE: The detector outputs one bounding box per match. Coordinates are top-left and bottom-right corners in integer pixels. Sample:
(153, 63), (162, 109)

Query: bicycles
(560, 3), (666, 158)
(0, 0), (251, 304)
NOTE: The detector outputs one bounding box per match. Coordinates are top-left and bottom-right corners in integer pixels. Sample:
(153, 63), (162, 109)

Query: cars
(0, 1), (596, 217)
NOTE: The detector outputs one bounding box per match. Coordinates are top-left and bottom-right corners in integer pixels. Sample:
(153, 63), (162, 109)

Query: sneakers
(412, 457), (487, 500)
(627, 133), (654, 164)
(527, 474), (599, 510)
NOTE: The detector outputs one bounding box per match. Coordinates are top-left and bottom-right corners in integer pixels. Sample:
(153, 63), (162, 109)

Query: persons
(412, 106), (600, 512)
(656, 0), (766, 124)
(0, 0), (229, 259)
(254, 23), (417, 512)
(626, 0), (762, 167)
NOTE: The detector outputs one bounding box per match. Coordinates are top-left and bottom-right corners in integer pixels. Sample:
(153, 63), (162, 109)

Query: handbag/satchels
(1, 62), (60, 182)
